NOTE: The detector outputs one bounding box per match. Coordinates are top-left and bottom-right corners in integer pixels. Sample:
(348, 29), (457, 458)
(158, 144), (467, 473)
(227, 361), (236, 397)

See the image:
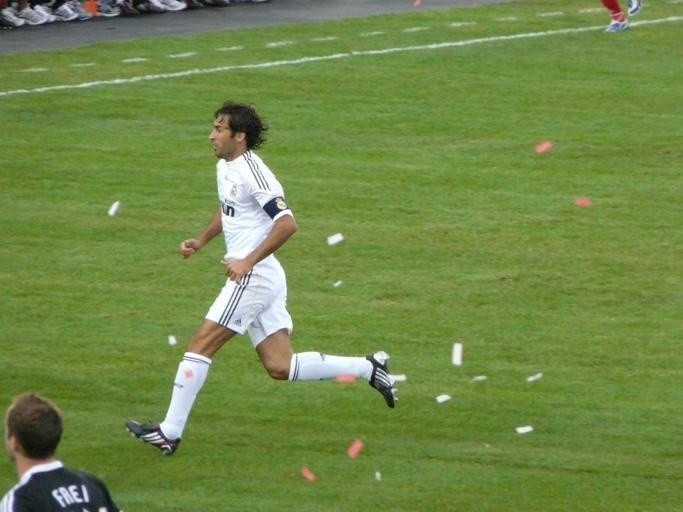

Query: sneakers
(0, 0), (230, 27)
(605, 19), (629, 32)
(126, 420), (180, 455)
(628, 0), (640, 16)
(366, 351), (394, 408)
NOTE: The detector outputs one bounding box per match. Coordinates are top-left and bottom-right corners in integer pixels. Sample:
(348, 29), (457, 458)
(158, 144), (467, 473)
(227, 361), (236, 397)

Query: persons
(601, 0), (645, 34)
(123, 99), (401, 456)
(0, 0), (233, 29)
(0, 391), (121, 512)
